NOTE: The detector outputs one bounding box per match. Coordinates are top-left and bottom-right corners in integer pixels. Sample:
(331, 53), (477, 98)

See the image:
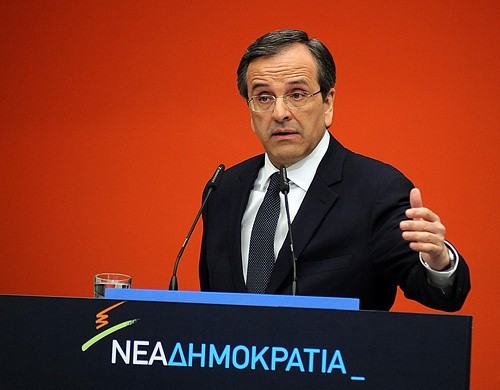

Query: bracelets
(421, 247), (454, 271)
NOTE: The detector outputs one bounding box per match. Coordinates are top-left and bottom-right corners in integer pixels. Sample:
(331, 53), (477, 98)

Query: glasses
(247, 90), (323, 111)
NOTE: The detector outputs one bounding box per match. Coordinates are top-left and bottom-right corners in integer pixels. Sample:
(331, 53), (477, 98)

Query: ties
(245, 170), (283, 294)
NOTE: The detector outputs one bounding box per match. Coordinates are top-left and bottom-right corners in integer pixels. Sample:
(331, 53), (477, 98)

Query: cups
(93, 273), (133, 300)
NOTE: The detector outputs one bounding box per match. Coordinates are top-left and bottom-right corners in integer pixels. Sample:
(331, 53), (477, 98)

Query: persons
(198, 28), (472, 311)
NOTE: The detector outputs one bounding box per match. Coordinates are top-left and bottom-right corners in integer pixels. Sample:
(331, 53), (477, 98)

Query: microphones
(169, 164), (225, 291)
(279, 165), (296, 296)
